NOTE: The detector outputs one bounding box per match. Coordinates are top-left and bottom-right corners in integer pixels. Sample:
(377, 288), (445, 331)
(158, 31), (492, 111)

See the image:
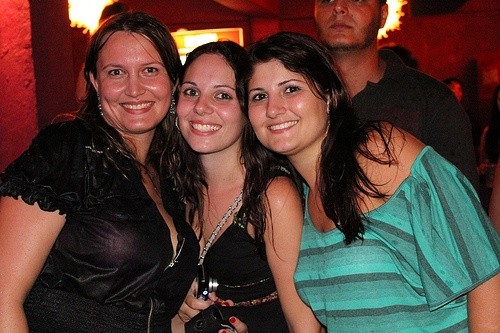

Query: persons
(313, 0), (469, 176)
(49, 3), (132, 126)
(0, 10), (500, 333)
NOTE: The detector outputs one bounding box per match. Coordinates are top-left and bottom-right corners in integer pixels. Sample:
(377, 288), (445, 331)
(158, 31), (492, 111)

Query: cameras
(184, 305), (239, 333)
